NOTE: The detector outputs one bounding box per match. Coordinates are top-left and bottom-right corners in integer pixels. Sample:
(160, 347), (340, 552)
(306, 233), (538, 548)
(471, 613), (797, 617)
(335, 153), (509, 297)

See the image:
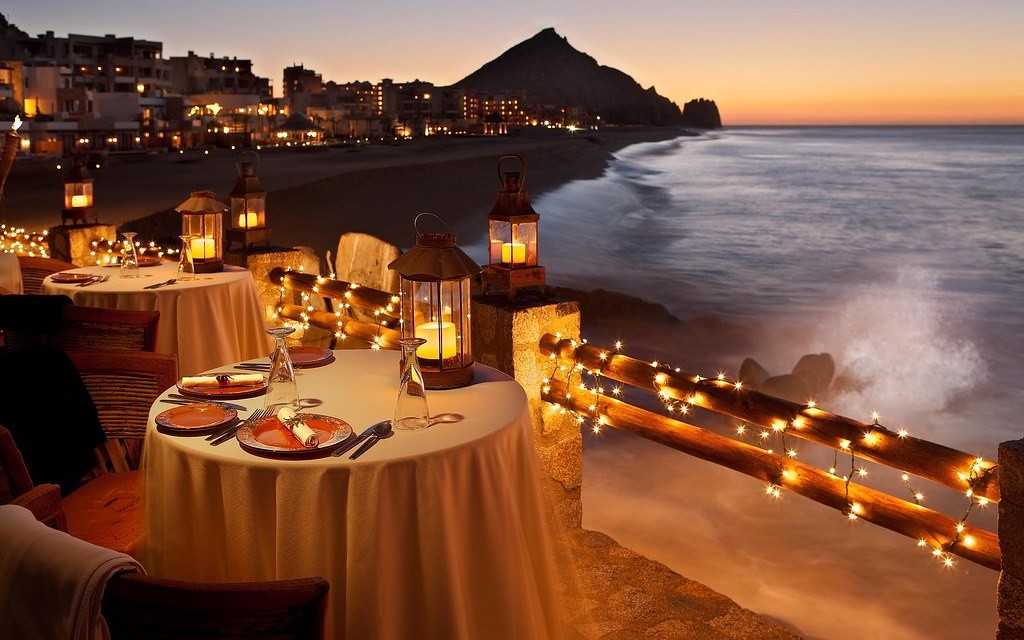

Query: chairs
(0, 255), (81, 296)
(0, 343), (187, 558)
(0, 479), (331, 640)
(0, 302), (162, 351)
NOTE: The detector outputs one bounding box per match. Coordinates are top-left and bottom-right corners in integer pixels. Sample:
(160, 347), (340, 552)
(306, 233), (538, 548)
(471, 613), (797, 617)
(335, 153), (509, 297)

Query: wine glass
(263, 328), (300, 415)
(177, 235), (195, 282)
(394, 338), (430, 431)
(120, 232), (140, 278)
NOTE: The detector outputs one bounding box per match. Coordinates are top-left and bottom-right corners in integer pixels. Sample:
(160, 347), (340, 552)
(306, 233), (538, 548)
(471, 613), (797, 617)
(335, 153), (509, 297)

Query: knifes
(332, 419), (392, 457)
(168, 393), (247, 411)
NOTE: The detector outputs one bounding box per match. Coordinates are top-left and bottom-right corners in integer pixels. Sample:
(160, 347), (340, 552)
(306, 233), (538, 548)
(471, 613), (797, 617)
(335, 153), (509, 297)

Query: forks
(81, 274), (112, 286)
(205, 409), (263, 442)
(211, 406), (276, 446)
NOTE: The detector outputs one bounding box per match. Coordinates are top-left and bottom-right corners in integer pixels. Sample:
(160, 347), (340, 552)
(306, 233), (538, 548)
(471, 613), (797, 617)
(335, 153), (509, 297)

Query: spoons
(349, 422), (392, 461)
(152, 279), (176, 289)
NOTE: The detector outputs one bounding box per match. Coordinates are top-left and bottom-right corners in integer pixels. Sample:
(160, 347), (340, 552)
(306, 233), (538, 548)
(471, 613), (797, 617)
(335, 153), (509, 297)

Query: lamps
(224, 148), (274, 252)
(177, 187), (225, 272)
(388, 210), (484, 388)
(478, 153), (551, 297)
(59, 150), (102, 225)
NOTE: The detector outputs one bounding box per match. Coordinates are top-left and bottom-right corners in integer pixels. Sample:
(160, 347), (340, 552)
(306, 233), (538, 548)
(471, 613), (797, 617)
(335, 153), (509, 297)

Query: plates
(50, 274), (93, 282)
(155, 403), (238, 432)
(137, 258), (160, 266)
(268, 346), (333, 367)
(176, 372), (268, 397)
(236, 412), (352, 451)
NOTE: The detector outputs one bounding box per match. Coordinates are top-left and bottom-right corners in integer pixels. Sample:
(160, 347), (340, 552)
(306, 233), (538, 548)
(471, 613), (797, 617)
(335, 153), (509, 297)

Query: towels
(0, 341), (107, 498)
(0, 504), (148, 640)
(0, 295), (75, 339)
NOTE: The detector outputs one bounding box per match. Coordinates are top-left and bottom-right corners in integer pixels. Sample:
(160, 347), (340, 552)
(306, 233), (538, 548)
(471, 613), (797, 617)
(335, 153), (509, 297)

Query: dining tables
(41, 259), (274, 379)
(139, 348), (584, 640)
